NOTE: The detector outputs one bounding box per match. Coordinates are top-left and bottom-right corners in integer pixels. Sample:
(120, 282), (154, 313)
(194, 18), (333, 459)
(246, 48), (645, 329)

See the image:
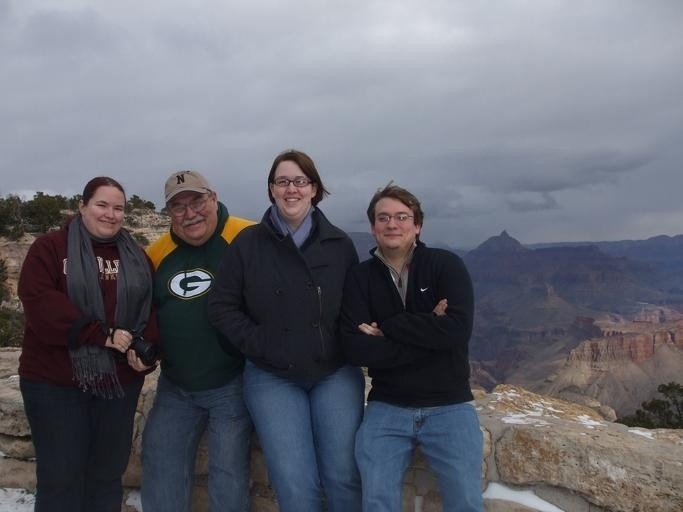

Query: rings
(120, 329), (125, 335)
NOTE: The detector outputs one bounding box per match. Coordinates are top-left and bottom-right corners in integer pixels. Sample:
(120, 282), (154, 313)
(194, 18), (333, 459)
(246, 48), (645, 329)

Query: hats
(163, 169), (213, 202)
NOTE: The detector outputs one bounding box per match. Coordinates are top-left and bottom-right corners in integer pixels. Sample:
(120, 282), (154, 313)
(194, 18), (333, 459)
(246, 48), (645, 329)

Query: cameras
(118, 330), (158, 365)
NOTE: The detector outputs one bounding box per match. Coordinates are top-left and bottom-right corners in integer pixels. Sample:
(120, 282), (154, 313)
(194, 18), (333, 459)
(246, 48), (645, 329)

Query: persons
(336, 185), (484, 511)
(139, 170), (258, 511)
(210, 150), (366, 511)
(17, 177), (160, 512)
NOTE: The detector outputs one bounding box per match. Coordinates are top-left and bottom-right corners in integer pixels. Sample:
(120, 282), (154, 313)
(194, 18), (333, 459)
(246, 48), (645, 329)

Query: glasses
(374, 212), (415, 221)
(271, 175), (315, 189)
(168, 200), (211, 215)
